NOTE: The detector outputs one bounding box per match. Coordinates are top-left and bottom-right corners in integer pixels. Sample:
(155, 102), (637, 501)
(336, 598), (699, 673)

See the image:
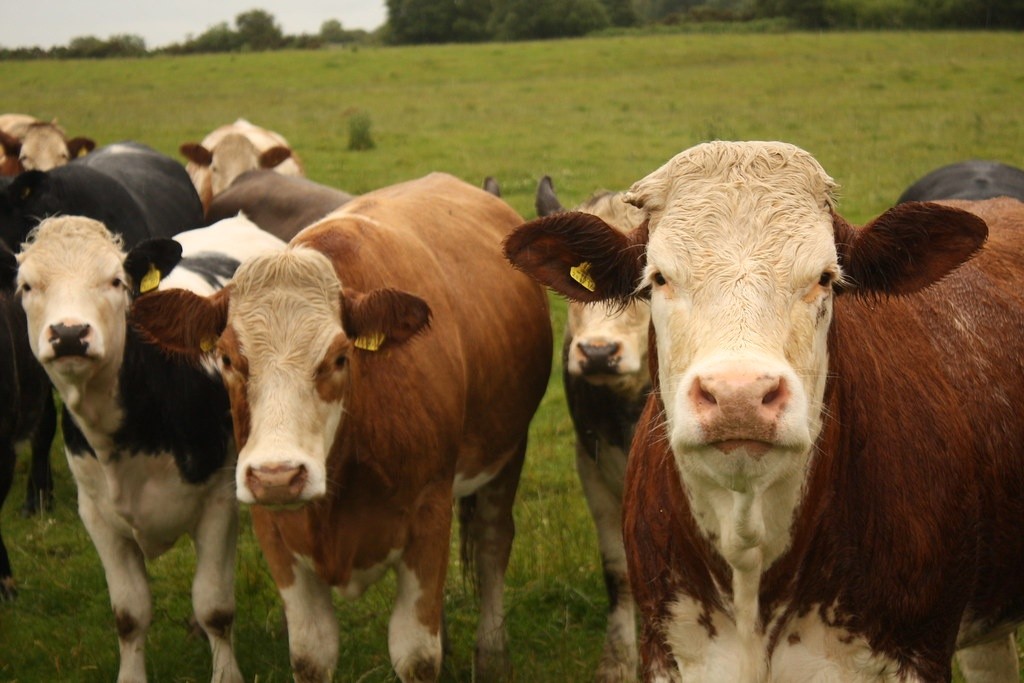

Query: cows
(0, 107), (552, 683)
(502, 137), (1024, 683)
